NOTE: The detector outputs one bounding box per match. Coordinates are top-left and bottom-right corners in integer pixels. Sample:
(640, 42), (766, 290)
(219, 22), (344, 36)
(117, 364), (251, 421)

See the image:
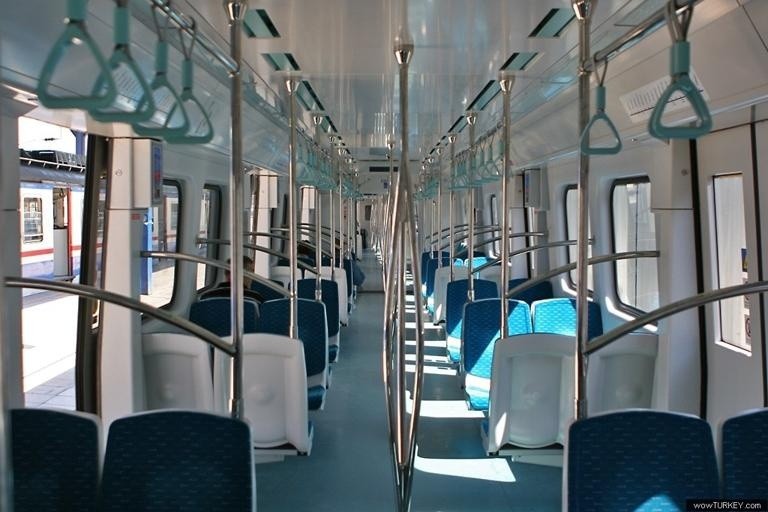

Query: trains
(16, 149), (213, 297)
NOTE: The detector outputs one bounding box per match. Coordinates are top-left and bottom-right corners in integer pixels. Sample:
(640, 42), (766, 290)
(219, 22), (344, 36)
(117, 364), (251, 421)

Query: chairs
(561, 408), (721, 512)
(717, 407), (768, 512)
(6, 406), (104, 512)
(96, 406), (257, 512)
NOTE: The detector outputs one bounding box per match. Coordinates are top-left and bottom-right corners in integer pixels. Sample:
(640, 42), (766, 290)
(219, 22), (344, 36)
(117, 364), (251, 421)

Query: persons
(323, 239), (365, 287)
(201, 253), (266, 303)
(297, 240), (314, 265)
